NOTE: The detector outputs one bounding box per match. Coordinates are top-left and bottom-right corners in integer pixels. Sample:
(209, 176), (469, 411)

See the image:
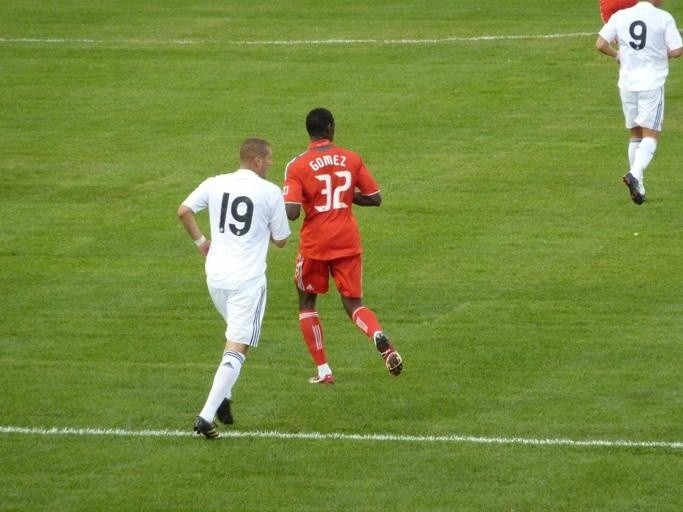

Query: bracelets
(194, 236), (206, 246)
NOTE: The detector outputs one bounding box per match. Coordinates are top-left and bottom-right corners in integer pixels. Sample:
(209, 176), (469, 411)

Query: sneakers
(622, 173), (643, 205)
(193, 416), (219, 438)
(374, 331), (403, 376)
(308, 374), (334, 385)
(217, 398), (233, 424)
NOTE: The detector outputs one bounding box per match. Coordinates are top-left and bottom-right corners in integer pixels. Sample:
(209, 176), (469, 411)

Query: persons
(600, 0), (638, 24)
(282, 108), (404, 384)
(596, 0), (683, 204)
(178, 138), (292, 439)
